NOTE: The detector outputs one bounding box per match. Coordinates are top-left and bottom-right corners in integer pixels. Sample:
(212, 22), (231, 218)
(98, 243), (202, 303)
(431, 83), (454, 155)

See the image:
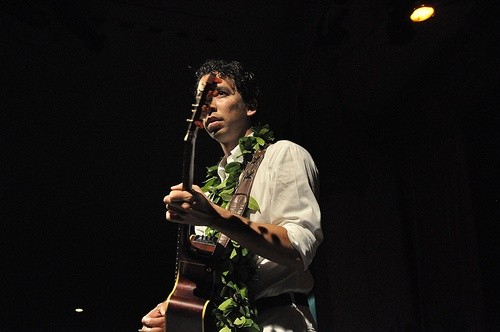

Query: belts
(253, 291), (309, 312)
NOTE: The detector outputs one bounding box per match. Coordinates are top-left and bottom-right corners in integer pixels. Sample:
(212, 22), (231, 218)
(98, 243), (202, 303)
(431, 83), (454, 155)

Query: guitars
(160, 70), (225, 332)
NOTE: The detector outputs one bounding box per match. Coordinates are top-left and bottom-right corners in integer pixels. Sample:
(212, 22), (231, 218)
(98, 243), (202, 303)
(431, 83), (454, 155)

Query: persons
(137, 60), (325, 332)
